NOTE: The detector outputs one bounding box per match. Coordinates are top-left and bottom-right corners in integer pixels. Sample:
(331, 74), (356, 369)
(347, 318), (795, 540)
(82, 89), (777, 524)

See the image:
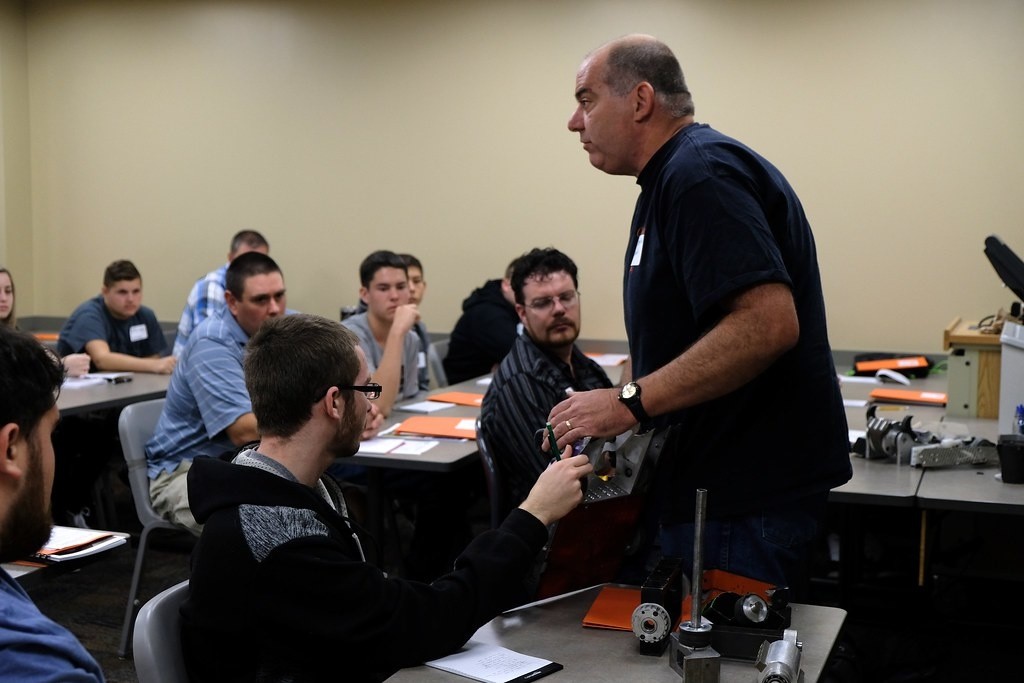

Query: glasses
(522, 287), (581, 314)
(320, 383), (382, 399)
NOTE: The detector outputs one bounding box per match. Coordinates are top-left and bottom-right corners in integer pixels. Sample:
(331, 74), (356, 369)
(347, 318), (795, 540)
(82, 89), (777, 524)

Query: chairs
(132, 578), (194, 682)
(117, 394), (193, 660)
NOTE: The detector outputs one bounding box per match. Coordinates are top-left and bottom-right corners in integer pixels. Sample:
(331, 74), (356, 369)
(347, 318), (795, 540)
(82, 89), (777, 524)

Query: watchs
(617, 381), (653, 426)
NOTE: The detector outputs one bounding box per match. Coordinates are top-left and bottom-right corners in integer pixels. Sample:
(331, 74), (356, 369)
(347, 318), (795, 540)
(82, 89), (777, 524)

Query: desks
(0, 352), (1024, 683)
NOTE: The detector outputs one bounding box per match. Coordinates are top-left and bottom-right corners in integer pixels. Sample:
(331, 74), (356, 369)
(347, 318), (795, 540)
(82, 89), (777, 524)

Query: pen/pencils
(546, 423), (561, 461)
(385, 443), (405, 455)
(1016, 405), (1024, 435)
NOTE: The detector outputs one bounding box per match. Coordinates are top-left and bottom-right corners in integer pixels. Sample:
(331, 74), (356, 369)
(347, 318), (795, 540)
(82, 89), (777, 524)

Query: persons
(0, 230), (614, 599)
(541, 34), (854, 602)
(0, 327), (105, 683)
(179, 315), (592, 683)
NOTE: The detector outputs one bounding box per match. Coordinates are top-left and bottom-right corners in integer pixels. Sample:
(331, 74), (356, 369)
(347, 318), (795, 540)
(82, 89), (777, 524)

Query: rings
(565, 421), (572, 431)
